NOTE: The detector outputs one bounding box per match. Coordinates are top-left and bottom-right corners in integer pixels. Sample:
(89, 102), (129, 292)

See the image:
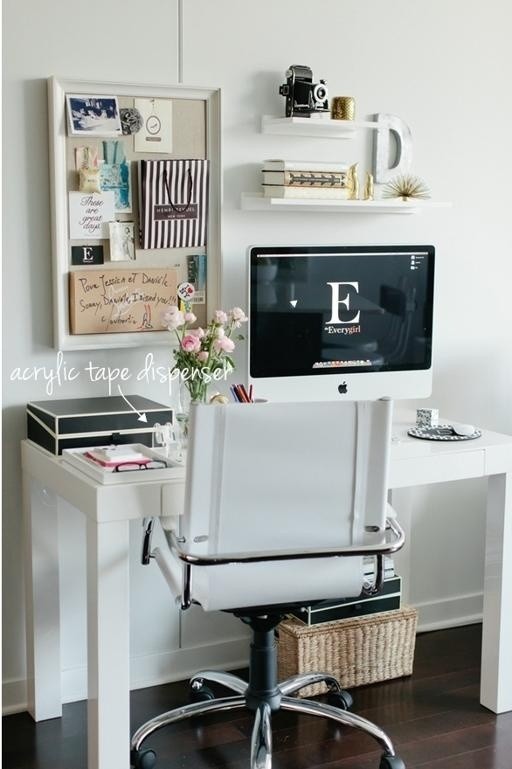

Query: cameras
(280, 64), (332, 119)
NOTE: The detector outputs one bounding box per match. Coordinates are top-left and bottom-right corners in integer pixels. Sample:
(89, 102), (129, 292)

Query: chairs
(130, 395), (409, 769)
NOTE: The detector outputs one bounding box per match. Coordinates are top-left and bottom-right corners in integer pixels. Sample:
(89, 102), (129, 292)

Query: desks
(18, 410), (511, 769)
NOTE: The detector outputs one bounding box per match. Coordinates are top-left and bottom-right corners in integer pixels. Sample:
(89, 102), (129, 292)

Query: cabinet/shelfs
(242, 112), (430, 215)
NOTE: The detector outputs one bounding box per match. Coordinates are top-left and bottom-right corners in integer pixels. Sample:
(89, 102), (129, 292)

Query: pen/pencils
(229, 383), (254, 402)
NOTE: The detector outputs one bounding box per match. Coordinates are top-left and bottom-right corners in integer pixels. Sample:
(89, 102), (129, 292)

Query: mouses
(451, 422), (476, 436)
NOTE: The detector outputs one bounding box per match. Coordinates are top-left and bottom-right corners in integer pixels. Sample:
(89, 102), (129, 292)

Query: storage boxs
(293, 576), (402, 625)
(26, 396), (172, 456)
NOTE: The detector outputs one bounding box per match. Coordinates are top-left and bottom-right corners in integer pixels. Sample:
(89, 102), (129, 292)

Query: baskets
(272, 606), (419, 698)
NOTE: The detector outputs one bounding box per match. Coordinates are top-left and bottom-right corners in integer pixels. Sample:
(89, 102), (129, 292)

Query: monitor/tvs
(247, 242), (438, 402)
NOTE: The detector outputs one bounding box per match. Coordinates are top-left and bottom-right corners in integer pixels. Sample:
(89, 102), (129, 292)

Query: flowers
(155, 307), (249, 400)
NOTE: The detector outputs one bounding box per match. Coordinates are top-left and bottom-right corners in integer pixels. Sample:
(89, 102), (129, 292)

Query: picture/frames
(48, 73), (224, 354)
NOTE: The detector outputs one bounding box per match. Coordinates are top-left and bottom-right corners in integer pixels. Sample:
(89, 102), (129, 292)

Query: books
(262, 184), (350, 200)
(262, 159), (346, 174)
(262, 170), (348, 188)
